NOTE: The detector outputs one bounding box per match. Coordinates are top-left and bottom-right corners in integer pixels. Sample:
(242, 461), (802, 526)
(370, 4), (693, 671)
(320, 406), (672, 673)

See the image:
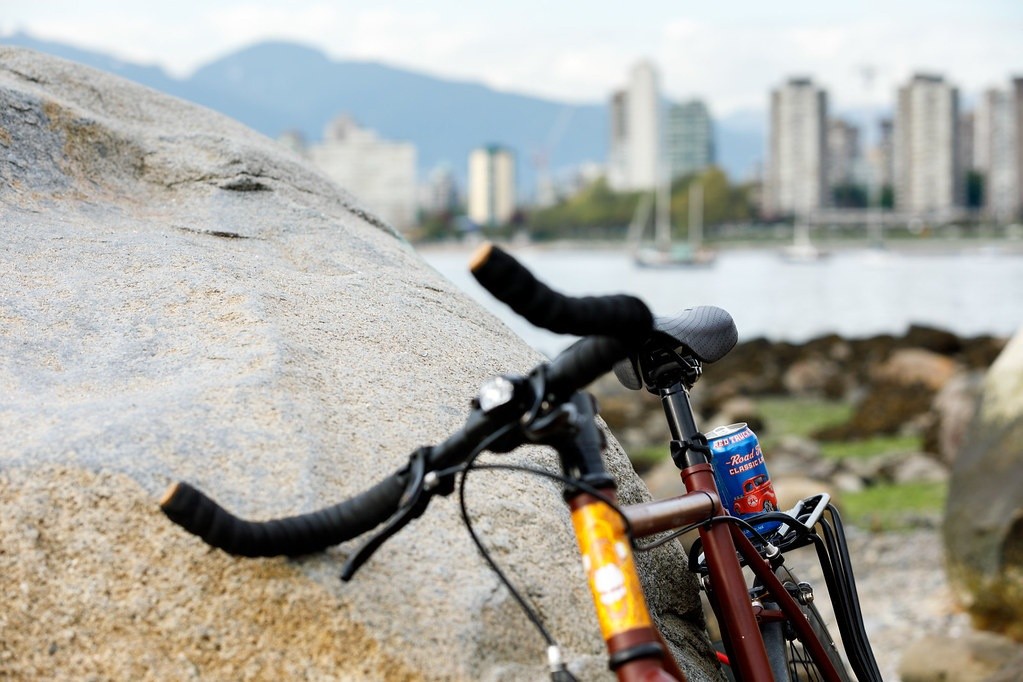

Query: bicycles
(157, 241), (885, 682)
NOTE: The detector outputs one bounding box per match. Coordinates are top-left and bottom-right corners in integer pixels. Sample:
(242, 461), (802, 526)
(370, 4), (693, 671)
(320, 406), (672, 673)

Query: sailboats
(781, 185), (830, 261)
(632, 164), (715, 267)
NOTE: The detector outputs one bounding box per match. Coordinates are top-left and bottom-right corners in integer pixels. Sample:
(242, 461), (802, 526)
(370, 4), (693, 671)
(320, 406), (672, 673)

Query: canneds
(702, 421), (785, 539)
